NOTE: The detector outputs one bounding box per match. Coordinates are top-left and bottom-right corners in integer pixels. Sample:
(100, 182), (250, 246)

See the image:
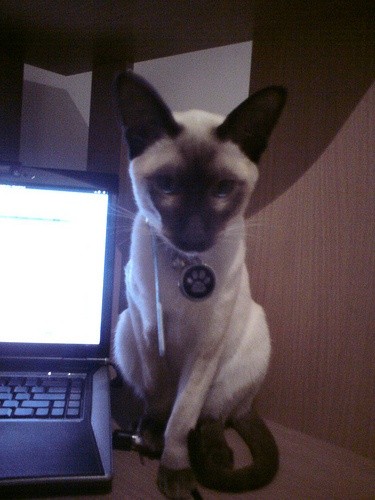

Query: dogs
(101, 63), (291, 500)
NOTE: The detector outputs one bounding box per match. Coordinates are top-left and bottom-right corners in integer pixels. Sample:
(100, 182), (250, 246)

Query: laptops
(0, 164), (113, 484)
